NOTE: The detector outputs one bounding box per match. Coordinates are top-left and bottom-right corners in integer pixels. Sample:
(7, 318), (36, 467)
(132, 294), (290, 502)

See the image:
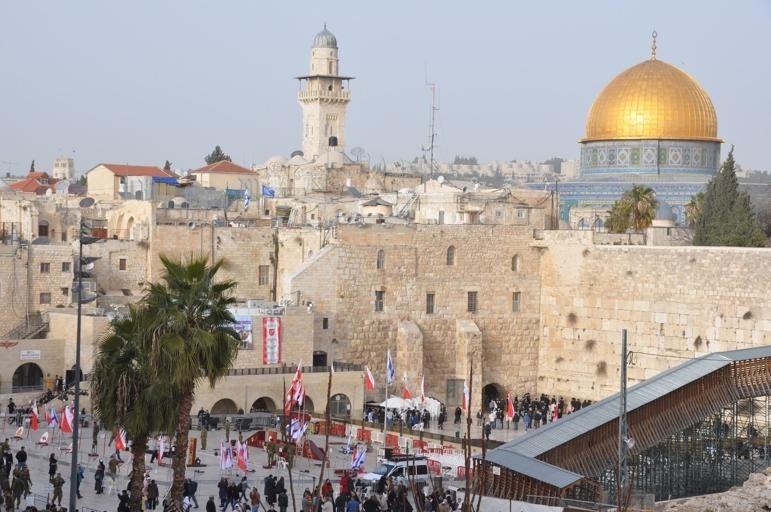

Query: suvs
(354, 453), (430, 497)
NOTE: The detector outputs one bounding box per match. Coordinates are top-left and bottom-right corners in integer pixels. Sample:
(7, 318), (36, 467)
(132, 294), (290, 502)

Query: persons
(225, 419), (230, 442)
(238, 431), (243, 442)
(198, 407), (209, 430)
(201, 425), (207, 450)
(453, 406), (462, 424)
(7, 373), (64, 428)
(439, 409), (445, 430)
(363, 404), (431, 430)
(476, 392), (592, 439)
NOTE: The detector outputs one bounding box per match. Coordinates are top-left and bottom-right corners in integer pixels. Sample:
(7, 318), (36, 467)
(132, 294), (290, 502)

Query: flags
(32, 400), (74, 431)
(116, 427), (127, 450)
(157, 434), (166, 466)
(400, 373), (413, 401)
(261, 183), (275, 198)
(347, 429), (367, 470)
(420, 375), (426, 402)
(284, 361), (308, 441)
(365, 367), (374, 391)
(244, 190), (249, 209)
(387, 351), (395, 385)
(462, 381), (469, 410)
